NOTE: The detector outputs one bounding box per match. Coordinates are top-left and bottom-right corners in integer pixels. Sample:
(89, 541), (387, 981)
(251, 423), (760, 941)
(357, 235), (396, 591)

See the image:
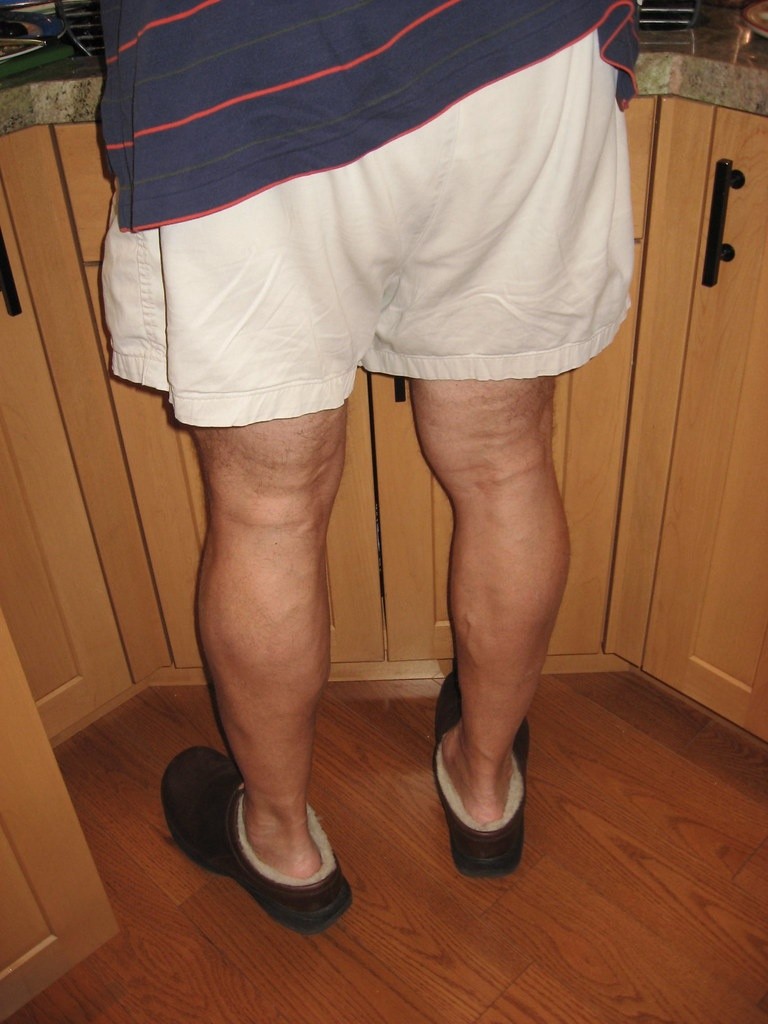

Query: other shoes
(433, 673), (529, 877)
(161, 745), (353, 932)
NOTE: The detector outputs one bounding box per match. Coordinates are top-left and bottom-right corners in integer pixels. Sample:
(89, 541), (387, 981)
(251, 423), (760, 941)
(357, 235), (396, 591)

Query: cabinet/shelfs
(1, 96), (767, 1024)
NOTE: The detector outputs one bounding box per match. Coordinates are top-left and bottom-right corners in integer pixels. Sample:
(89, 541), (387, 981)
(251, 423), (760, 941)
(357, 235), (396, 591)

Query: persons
(98, 2), (639, 928)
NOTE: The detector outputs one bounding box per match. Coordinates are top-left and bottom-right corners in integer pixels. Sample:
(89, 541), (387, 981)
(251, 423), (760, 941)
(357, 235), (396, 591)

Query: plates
(0, 11), (65, 63)
(743, 0), (768, 39)
